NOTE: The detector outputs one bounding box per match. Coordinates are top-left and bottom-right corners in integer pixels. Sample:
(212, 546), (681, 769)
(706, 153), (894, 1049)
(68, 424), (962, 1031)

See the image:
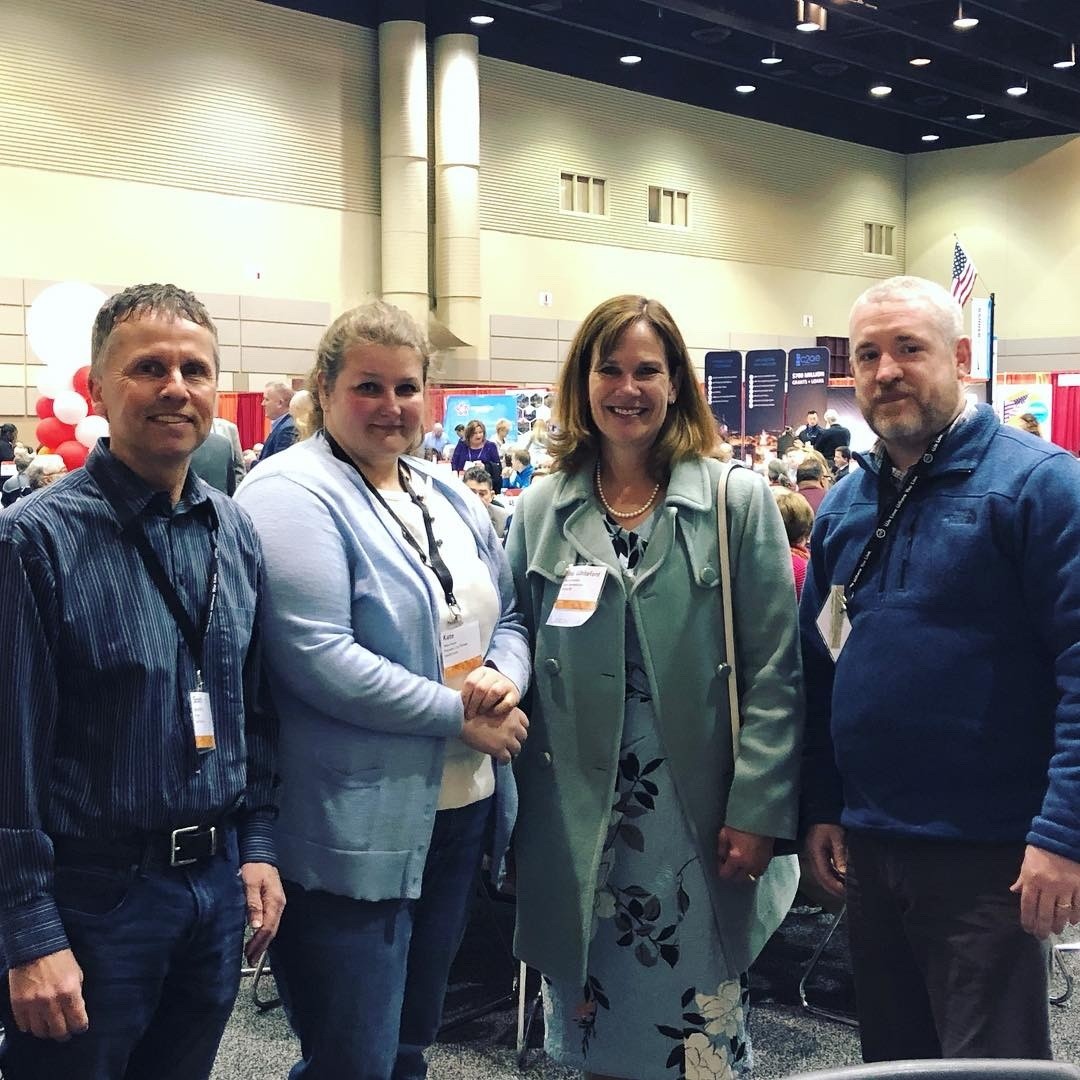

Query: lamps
(870, 80), (893, 96)
(966, 102), (985, 119)
(761, 45), (784, 65)
(795, 0), (827, 31)
(953, 3), (979, 28)
(1004, 80), (1028, 95)
(1052, 42), (1076, 69)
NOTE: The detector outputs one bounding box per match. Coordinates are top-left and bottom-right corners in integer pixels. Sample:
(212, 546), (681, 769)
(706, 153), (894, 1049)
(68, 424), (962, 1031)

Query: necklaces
(596, 459), (660, 518)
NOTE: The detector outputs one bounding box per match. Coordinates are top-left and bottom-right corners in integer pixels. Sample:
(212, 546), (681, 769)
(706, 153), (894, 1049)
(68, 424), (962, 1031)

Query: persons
(1009, 414), (1040, 437)
(0, 423), (69, 507)
(798, 276), (1080, 1070)
(233, 302), (534, 1080)
(720, 408), (851, 606)
(502, 297), (801, 1080)
(189, 377), (321, 498)
(421, 418), (561, 547)
(0, 282), (292, 1080)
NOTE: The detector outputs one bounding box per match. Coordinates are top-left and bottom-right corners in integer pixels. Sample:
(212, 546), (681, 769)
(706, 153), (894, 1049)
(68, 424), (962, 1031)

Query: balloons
(27, 281), (112, 472)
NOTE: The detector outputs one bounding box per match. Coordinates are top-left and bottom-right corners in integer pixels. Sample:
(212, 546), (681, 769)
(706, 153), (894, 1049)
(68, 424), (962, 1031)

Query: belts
(57, 826), (223, 868)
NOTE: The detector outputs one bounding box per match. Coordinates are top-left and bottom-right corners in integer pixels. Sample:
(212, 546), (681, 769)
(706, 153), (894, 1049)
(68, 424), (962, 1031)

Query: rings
(748, 874), (756, 880)
(1057, 903), (1071, 908)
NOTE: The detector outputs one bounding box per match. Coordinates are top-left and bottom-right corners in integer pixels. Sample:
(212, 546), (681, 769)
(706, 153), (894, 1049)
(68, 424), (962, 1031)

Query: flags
(950, 242), (977, 309)
